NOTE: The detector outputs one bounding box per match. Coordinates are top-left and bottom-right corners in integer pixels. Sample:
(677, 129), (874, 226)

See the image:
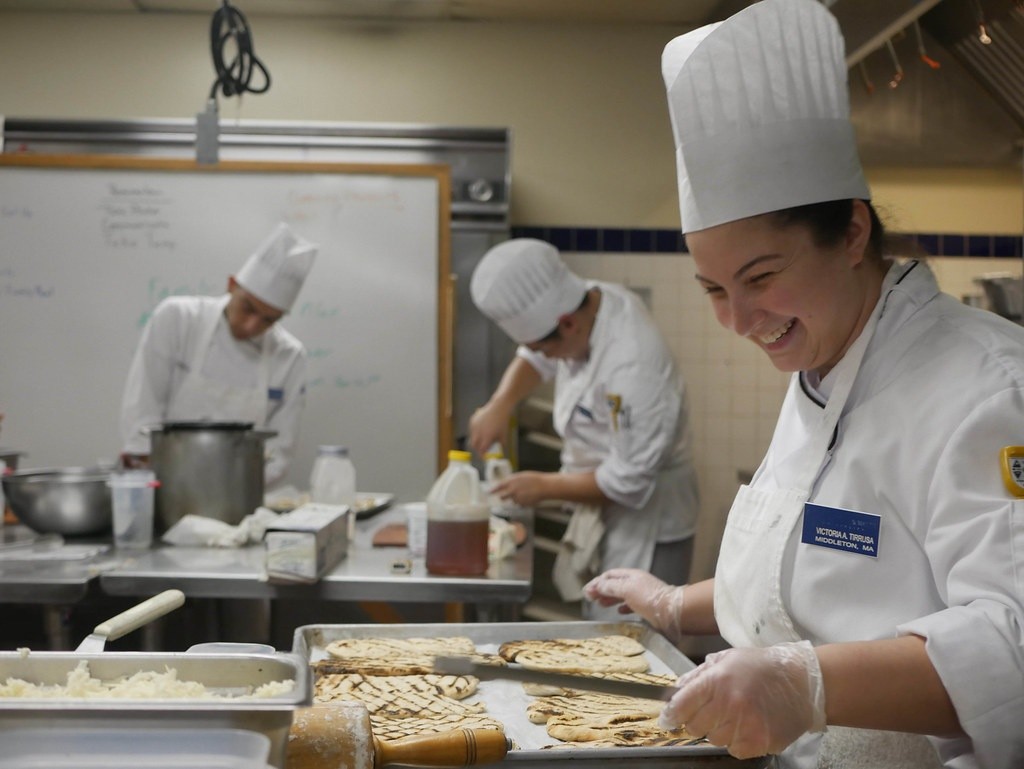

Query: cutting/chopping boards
(372, 520), (529, 546)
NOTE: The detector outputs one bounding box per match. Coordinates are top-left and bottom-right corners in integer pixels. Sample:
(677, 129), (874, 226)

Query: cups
(406, 501), (427, 555)
(105, 468), (157, 549)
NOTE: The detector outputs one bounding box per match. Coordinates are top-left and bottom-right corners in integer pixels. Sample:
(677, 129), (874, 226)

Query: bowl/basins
(0, 465), (112, 538)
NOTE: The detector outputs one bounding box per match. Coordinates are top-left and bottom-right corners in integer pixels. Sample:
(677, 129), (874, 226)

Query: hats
(661, 1), (875, 232)
(467, 237), (587, 348)
(237, 222), (319, 313)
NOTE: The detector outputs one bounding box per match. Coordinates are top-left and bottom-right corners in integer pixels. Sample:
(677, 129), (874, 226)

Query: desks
(0, 505), (533, 652)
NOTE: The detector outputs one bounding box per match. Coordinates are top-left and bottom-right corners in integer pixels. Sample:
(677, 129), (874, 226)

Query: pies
(313, 634), (715, 750)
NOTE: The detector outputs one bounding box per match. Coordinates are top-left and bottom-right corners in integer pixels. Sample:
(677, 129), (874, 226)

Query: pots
(138, 418), (281, 532)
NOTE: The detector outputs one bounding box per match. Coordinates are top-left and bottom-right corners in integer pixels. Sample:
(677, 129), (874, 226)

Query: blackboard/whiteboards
(1, 151), (454, 539)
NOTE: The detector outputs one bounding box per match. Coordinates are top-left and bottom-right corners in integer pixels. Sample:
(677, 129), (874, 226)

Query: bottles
(424, 448), (492, 577)
(307, 442), (357, 541)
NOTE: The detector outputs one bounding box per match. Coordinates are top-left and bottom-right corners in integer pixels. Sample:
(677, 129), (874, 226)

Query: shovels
(73, 586), (186, 652)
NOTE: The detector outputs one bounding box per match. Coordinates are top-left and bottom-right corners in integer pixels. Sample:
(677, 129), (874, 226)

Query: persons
(467, 240), (701, 602)
(584, 0), (1024, 769)
(118, 223), (319, 487)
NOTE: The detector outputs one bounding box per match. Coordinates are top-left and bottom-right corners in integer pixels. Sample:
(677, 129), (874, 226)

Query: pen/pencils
(576, 405), (593, 421)
